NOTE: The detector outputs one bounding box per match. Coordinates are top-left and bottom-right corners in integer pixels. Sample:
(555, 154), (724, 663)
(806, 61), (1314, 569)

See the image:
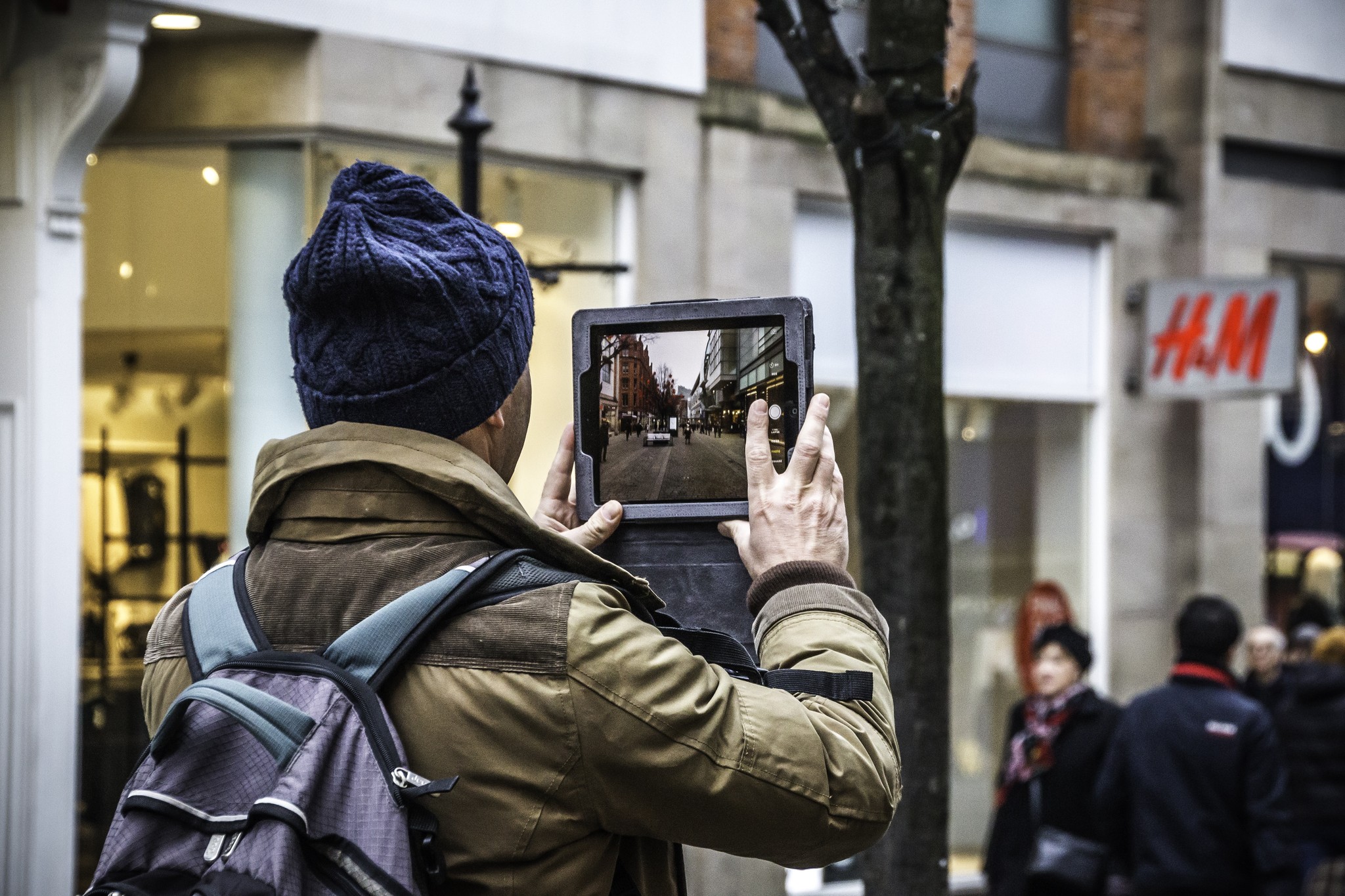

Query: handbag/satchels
(1025, 830), (1116, 892)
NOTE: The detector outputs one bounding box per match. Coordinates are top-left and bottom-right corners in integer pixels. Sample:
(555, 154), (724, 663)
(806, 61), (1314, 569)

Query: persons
(1098, 594), (1288, 896)
(980, 624), (1123, 896)
(600, 414), (748, 462)
(140, 161), (899, 896)
(1243, 591), (1345, 896)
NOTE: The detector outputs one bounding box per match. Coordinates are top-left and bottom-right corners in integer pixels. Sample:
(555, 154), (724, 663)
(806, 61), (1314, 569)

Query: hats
(283, 161), (533, 437)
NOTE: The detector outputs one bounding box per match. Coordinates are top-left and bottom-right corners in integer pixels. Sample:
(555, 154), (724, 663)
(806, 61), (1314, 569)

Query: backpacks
(82, 545), (604, 896)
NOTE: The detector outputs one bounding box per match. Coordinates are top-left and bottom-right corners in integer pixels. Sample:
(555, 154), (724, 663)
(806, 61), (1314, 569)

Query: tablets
(572, 294), (814, 523)
